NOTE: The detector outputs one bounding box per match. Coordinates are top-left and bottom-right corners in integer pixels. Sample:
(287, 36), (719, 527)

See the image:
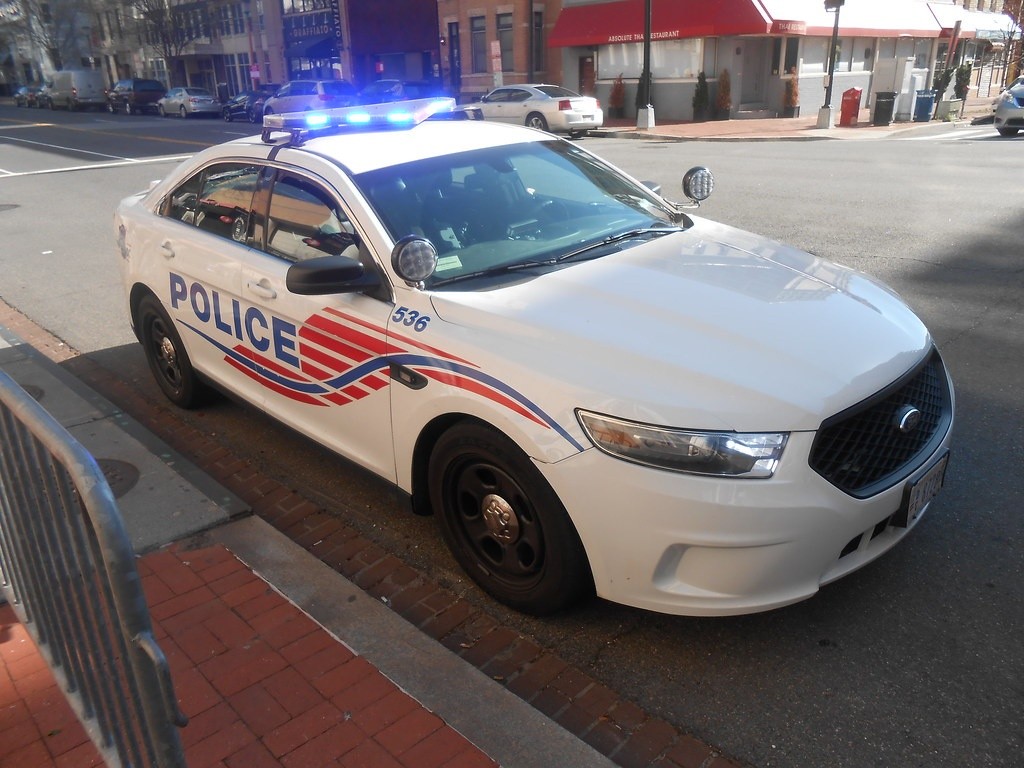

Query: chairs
(463, 160), (530, 234)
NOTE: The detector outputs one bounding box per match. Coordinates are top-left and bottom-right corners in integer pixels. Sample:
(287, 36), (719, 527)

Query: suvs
(110, 77), (167, 116)
(344, 76), (446, 108)
(222, 82), (280, 122)
(263, 78), (358, 121)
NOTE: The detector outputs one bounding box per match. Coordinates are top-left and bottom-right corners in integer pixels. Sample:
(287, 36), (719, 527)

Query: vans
(39, 69), (108, 113)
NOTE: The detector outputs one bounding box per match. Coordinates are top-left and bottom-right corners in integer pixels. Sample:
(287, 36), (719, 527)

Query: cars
(453, 82), (603, 139)
(113, 99), (956, 621)
(993, 74), (1024, 138)
(14, 87), (40, 109)
(156, 86), (224, 120)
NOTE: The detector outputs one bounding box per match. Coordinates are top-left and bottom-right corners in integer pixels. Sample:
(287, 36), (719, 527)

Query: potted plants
(607, 72), (626, 119)
(635, 69), (654, 119)
(692, 72), (709, 121)
(783, 67), (801, 118)
(716, 68), (730, 120)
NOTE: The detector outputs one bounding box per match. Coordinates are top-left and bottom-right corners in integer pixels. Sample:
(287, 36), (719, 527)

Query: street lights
(816, 0), (846, 129)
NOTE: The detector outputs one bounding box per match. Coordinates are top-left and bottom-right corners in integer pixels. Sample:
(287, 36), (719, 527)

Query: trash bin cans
(913, 89), (938, 122)
(840, 87), (863, 127)
(874, 92), (899, 126)
